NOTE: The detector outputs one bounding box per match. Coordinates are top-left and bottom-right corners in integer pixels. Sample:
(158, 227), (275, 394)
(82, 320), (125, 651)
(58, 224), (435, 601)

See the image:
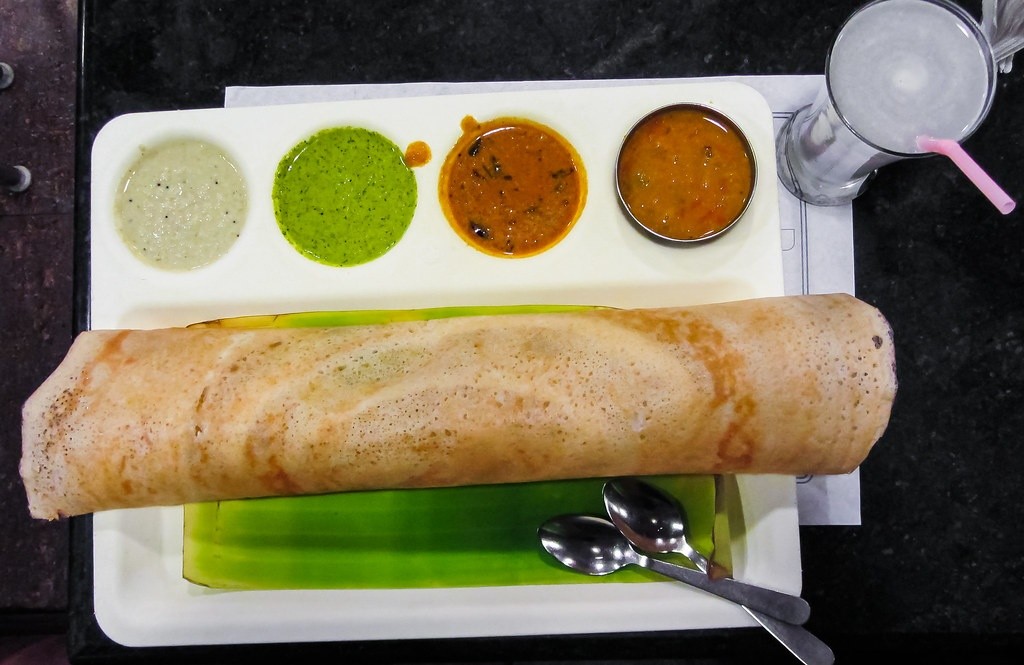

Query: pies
(19, 292), (899, 520)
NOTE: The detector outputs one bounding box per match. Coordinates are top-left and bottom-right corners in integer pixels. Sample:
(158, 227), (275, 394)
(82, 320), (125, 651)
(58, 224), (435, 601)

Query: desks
(65, 0), (1024, 665)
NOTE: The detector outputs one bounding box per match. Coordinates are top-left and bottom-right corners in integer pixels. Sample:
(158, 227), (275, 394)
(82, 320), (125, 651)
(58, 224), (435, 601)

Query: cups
(776, 0), (996, 208)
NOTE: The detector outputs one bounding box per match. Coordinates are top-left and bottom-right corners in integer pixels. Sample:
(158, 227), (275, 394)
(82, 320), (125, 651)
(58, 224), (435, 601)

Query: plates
(89, 82), (803, 648)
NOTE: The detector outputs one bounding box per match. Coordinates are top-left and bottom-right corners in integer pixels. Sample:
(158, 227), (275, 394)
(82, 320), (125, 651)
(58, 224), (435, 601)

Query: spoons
(601, 476), (835, 665)
(536, 513), (811, 626)
